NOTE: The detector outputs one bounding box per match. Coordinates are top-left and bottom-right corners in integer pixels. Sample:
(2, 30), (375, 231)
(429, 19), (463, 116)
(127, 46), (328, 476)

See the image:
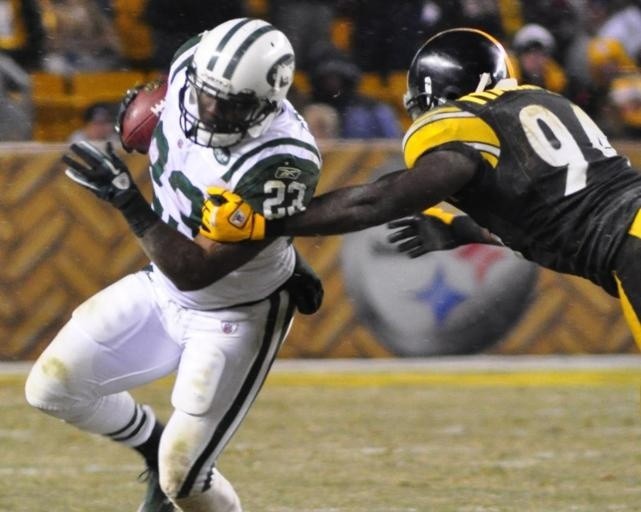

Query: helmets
(402, 28), (515, 123)
(177, 16), (295, 149)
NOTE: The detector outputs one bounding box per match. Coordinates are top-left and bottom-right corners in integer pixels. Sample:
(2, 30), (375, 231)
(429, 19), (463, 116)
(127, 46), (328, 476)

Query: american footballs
(121, 80), (170, 147)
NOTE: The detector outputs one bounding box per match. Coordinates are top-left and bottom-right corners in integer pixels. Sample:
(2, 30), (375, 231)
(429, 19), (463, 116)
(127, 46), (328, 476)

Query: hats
(511, 21), (555, 49)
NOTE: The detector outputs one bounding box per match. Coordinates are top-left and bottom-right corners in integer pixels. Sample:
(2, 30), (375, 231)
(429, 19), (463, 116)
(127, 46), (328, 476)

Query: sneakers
(134, 461), (171, 511)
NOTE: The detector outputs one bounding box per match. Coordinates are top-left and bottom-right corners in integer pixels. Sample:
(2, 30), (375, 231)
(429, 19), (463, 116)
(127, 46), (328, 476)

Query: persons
(195, 30), (641, 352)
(25, 16), (322, 512)
(2, 2), (641, 141)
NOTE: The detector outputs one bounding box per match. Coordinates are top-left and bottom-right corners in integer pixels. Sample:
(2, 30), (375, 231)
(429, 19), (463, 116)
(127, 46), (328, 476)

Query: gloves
(388, 207), (465, 260)
(61, 139), (138, 208)
